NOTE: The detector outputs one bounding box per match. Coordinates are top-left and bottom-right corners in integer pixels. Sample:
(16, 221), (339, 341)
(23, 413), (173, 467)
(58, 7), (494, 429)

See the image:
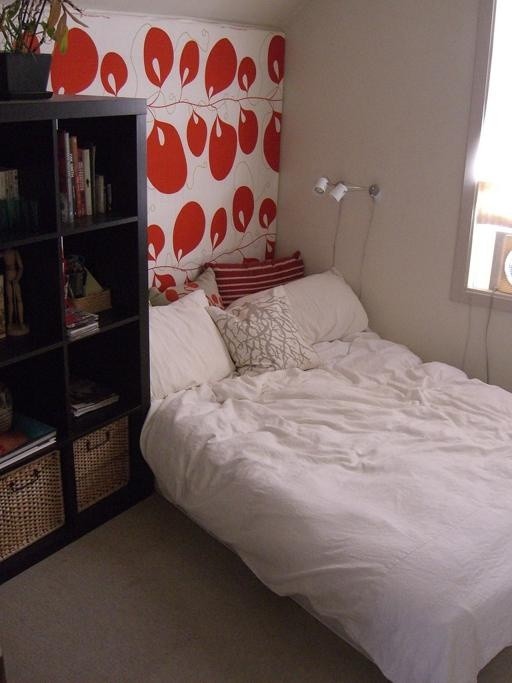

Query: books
(70, 384), (120, 418)
(1, 168), (23, 232)
(0, 413), (58, 469)
(64, 305), (100, 342)
(56, 129), (118, 224)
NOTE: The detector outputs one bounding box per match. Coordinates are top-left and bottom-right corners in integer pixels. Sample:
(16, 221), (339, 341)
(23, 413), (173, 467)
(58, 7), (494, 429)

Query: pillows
(150, 248), (372, 405)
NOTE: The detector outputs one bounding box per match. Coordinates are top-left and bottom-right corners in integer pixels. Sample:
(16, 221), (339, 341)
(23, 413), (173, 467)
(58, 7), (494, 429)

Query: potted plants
(0, 0), (90, 95)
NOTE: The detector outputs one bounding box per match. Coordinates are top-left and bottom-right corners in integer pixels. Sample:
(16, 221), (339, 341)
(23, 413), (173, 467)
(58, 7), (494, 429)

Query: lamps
(313, 175), (382, 204)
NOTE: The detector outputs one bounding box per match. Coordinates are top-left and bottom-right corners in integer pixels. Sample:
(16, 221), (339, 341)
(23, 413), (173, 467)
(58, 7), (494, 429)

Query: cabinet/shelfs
(1, 96), (150, 582)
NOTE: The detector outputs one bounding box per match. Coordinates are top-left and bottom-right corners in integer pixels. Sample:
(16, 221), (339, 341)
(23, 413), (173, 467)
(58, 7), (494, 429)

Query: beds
(146, 328), (512, 683)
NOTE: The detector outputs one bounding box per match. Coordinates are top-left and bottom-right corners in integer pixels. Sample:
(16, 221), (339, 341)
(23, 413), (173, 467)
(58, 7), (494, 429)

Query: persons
(0, 246), (27, 331)
